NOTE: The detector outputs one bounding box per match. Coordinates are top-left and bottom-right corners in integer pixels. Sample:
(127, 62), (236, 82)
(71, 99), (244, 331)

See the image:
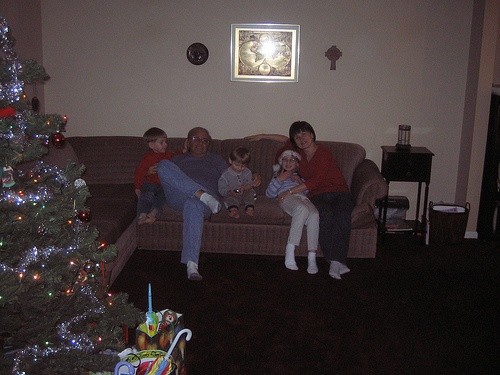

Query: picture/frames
(230, 23), (301, 82)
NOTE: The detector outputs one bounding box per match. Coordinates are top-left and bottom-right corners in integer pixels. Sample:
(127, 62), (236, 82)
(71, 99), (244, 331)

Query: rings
(281, 197), (283, 199)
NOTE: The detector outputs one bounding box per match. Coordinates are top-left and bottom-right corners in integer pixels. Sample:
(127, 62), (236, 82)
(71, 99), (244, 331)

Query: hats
(272, 139), (302, 172)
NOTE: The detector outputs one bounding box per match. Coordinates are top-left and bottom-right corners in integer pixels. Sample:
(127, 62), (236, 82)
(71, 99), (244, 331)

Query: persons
(147, 127), (261, 282)
(266, 149), (319, 274)
(276, 121), (352, 280)
(217, 146), (256, 219)
(134, 127), (189, 225)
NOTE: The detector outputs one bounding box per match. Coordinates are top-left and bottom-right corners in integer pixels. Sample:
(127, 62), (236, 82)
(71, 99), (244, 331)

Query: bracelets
(289, 188), (293, 195)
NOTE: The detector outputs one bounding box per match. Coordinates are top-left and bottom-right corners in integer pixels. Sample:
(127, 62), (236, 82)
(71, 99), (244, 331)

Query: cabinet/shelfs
(379, 145), (435, 247)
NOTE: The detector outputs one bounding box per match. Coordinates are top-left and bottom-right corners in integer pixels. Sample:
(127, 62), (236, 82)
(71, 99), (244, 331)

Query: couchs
(16, 135), (388, 300)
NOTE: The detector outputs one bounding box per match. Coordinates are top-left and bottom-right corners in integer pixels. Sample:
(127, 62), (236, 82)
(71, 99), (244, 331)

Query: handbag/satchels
(121, 349), (178, 375)
(135, 309), (188, 375)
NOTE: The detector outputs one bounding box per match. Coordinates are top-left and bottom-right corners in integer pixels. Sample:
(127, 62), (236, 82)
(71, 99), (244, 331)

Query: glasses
(189, 135), (210, 144)
(281, 156), (298, 163)
